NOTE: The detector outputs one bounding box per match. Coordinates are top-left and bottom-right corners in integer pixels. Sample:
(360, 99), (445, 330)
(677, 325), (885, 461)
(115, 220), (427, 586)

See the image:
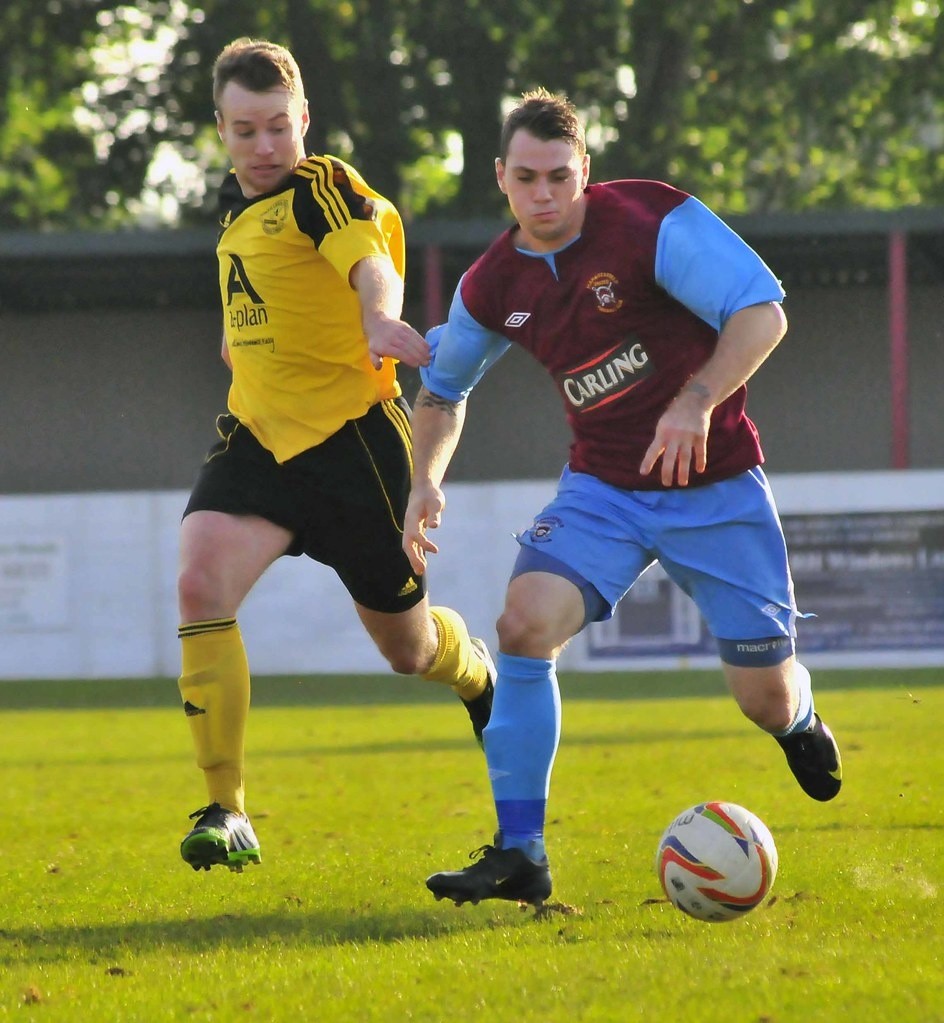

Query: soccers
(656, 801), (780, 924)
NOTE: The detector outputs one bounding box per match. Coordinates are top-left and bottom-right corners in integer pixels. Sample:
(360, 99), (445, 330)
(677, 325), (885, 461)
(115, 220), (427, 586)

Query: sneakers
(181, 802), (262, 873)
(423, 831), (552, 906)
(775, 711), (842, 802)
(461, 639), (497, 737)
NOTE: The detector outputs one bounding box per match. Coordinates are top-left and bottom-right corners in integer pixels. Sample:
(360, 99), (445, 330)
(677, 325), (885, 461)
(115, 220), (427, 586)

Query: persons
(402, 87), (843, 905)
(177, 37), (498, 873)
